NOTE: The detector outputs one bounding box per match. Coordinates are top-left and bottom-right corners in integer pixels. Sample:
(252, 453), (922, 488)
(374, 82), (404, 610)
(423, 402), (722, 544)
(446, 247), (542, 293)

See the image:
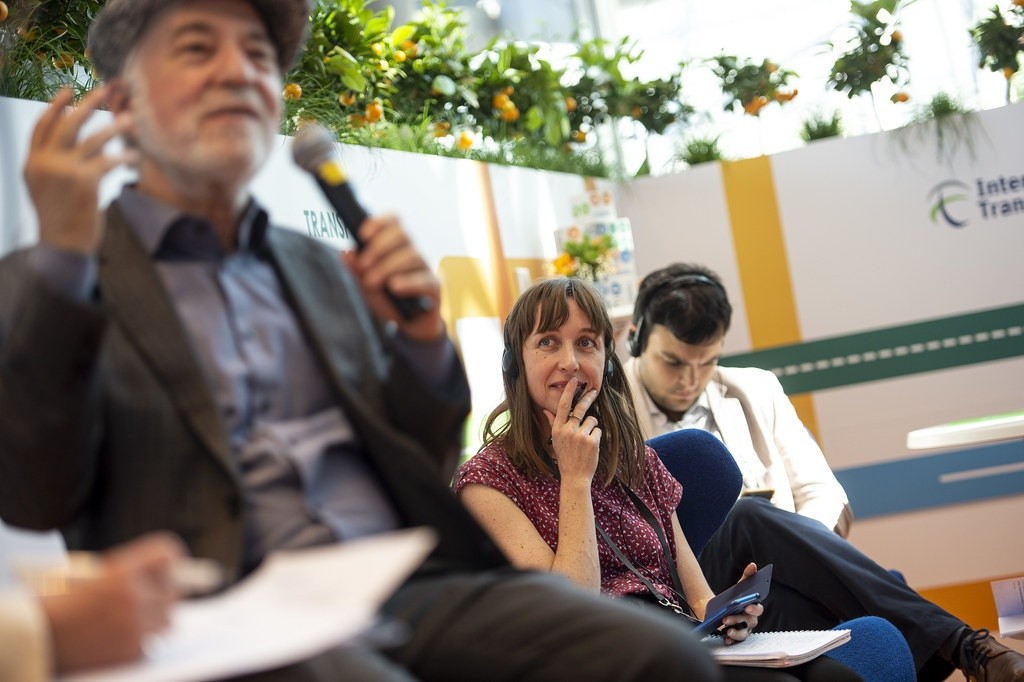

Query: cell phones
(691, 591), (761, 635)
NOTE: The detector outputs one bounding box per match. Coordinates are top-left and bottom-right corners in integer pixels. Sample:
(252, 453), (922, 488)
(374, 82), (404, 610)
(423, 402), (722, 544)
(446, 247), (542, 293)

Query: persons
(0, 529), (190, 682)
(622, 262), (1024, 682)
(0, 0), (722, 682)
(453, 276), (917, 682)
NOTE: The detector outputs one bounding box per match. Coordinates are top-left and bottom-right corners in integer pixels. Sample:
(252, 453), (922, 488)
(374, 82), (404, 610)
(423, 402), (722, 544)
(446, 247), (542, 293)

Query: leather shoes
(956, 629), (1024, 682)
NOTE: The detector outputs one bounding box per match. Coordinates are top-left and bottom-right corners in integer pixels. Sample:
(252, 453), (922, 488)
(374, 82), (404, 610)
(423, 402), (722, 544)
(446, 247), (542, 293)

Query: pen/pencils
(548, 380), (588, 446)
(14, 551), (227, 598)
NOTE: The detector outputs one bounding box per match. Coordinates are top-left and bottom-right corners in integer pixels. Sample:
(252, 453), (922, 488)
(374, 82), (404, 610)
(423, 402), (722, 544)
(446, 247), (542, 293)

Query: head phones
(624, 272), (721, 359)
(501, 308), (616, 384)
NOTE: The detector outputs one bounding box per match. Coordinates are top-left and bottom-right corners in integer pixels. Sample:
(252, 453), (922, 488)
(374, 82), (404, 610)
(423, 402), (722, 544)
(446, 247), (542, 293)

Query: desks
(908, 410), (1024, 450)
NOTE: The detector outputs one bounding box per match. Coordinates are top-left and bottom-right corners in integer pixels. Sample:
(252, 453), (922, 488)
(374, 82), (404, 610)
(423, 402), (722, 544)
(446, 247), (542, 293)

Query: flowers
(541, 230), (621, 282)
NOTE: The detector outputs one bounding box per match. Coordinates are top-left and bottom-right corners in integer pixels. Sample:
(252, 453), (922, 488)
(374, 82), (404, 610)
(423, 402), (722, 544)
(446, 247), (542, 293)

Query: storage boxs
(554, 189), (639, 308)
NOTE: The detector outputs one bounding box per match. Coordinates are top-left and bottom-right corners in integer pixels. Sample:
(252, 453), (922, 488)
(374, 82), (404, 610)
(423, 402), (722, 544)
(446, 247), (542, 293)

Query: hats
(86, 0), (312, 91)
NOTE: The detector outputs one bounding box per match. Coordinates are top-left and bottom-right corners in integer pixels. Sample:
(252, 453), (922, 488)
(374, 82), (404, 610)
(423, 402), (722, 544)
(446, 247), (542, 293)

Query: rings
(569, 412), (582, 421)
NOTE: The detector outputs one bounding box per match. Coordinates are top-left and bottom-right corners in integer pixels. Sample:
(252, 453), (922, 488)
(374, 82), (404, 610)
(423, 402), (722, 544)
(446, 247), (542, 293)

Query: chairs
(645, 428), (917, 682)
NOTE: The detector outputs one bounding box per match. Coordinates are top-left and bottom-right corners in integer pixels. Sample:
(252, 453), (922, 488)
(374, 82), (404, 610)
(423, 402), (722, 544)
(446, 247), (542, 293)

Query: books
(700, 629), (851, 667)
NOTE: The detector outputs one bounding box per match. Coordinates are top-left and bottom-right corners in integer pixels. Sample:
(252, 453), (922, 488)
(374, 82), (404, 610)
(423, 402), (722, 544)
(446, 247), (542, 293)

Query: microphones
(290, 121), (431, 322)
